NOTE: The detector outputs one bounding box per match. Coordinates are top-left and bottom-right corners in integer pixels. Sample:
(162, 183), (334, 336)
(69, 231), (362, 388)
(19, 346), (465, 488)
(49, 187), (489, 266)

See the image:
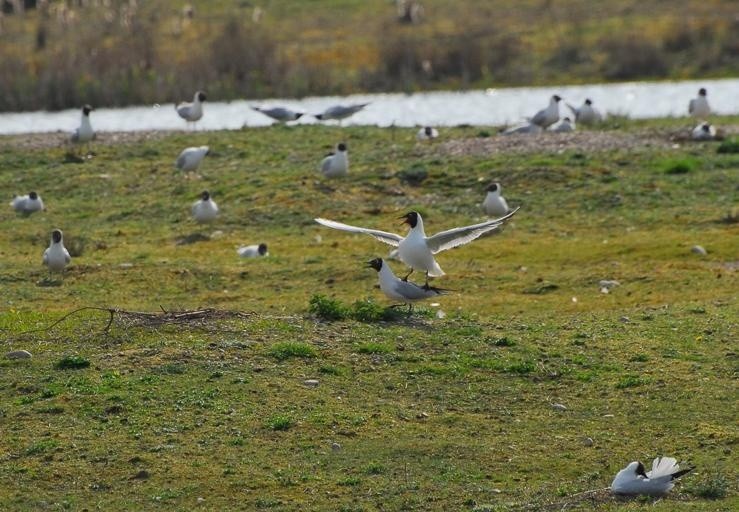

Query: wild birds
(418, 125), (439, 140)
(39, 227), (73, 281)
(320, 142), (348, 182)
(68, 104), (97, 161)
(480, 183), (510, 221)
(193, 190), (218, 233)
(172, 91), (211, 134)
(174, 144), (225, 179)
(9, 192), (46, 216)
(235, 242), (271, 260)
(687, 87), (718, 142)
(503, 92), (602, 135)
(314, 205), (523, 317)
(609, 451), (699, 497)
(247, 102), (372, 128)
